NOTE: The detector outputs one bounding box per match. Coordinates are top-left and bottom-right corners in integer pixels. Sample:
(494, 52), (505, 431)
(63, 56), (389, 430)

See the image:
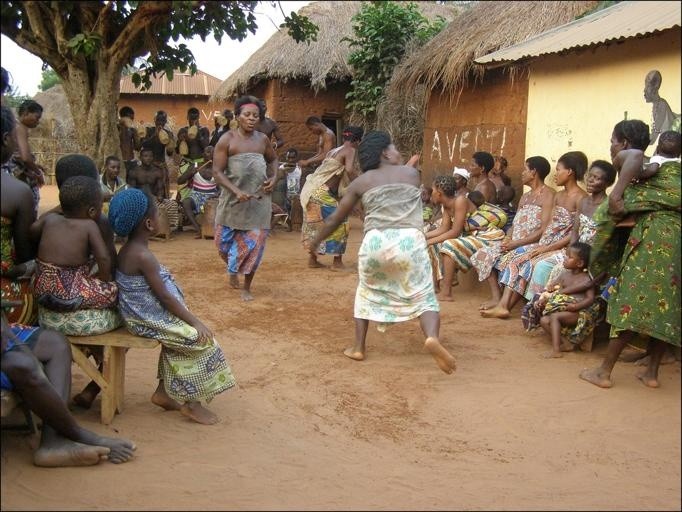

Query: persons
(210, 96), (279, 302)
(644, 69), (674, 136)
(308, 131), (459, 375)
(576, 121), (681, 390)
(479, 150), (617, 321)
(534, 241), (595, 359)
(97, 97), (336, 229)
(299, 125), (365, 269)
(423, 152), (516, 303)
(642, 128), (679, 177)
(106, 187), (237, 424)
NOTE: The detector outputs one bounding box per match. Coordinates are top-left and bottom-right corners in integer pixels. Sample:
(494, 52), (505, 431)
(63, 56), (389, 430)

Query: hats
(109, 188), (148, 237)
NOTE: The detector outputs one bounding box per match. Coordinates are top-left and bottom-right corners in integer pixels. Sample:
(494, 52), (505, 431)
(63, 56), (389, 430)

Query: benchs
(65, 326), (160, 428)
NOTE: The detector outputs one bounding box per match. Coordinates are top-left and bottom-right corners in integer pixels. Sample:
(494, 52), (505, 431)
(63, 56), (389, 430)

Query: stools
(0, 389), (39, 442)
